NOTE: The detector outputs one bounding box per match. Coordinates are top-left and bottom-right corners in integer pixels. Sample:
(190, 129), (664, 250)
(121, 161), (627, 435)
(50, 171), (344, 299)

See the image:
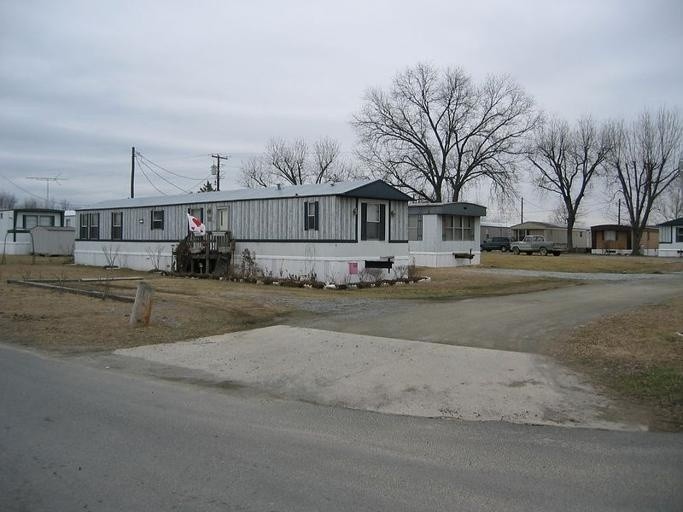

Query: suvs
(479, 236), (514, 252)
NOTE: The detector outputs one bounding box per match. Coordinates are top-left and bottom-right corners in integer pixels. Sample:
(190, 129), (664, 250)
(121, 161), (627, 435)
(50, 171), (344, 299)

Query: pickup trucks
(511, 235), (568, 255)
